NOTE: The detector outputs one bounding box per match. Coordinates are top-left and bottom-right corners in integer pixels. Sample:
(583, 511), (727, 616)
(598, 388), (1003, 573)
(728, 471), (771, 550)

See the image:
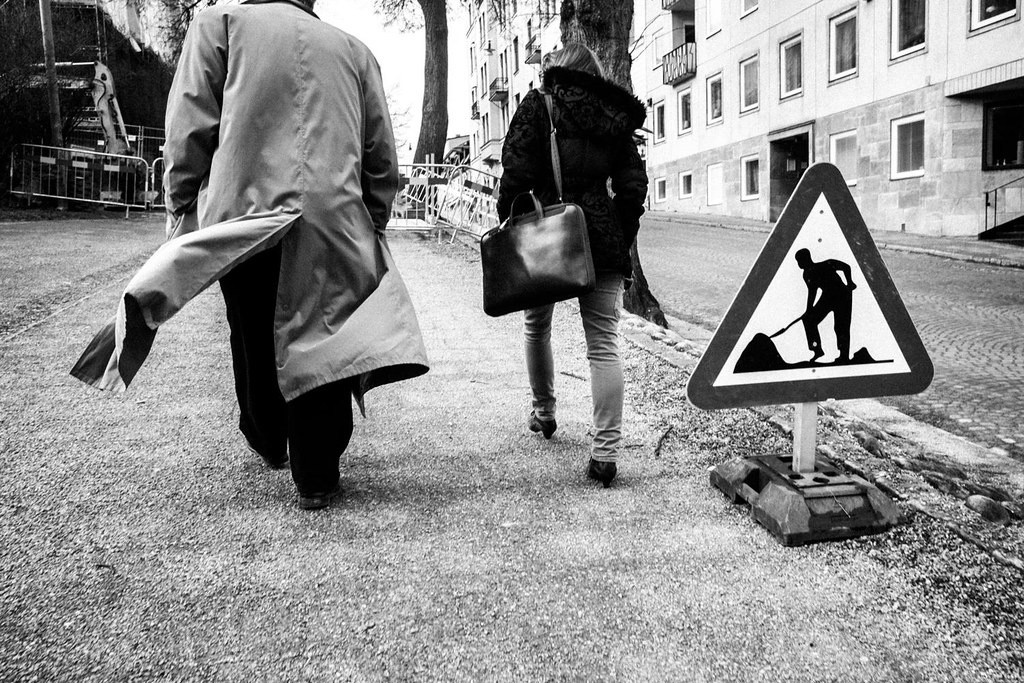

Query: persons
(498, 43), (649, 486)
(66, 0), (432, 510)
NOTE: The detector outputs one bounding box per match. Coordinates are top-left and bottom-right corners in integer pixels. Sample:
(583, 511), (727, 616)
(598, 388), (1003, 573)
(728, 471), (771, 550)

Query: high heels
(586, 456), (617, 488)
(527, 411), (557, 440)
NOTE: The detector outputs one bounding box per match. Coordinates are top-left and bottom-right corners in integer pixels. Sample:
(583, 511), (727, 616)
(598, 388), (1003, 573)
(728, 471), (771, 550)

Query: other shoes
(245, 442), (289, 470)
(299, 488), (344, 510)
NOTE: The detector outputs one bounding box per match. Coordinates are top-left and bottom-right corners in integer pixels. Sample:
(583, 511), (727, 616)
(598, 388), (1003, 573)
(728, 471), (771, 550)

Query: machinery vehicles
(0, 60), (161, 212)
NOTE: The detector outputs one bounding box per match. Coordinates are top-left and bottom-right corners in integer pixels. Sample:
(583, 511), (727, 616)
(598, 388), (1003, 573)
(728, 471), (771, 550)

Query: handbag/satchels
(480, 191), (597, 317)
